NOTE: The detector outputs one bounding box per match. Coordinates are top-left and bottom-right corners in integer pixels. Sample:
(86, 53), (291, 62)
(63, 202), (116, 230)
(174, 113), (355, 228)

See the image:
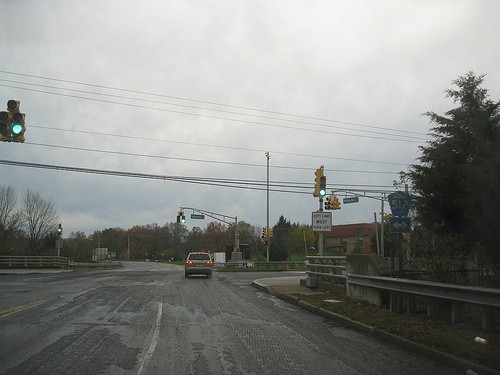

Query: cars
(185, 252), (214, 278)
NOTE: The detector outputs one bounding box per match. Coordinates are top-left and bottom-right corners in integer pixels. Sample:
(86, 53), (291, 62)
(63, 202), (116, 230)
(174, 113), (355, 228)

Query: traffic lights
(0, 100), (26, 143)
(58, 224), (63, 235)
(177, 211), (186, 223)
(313, 169), (326, 197)
